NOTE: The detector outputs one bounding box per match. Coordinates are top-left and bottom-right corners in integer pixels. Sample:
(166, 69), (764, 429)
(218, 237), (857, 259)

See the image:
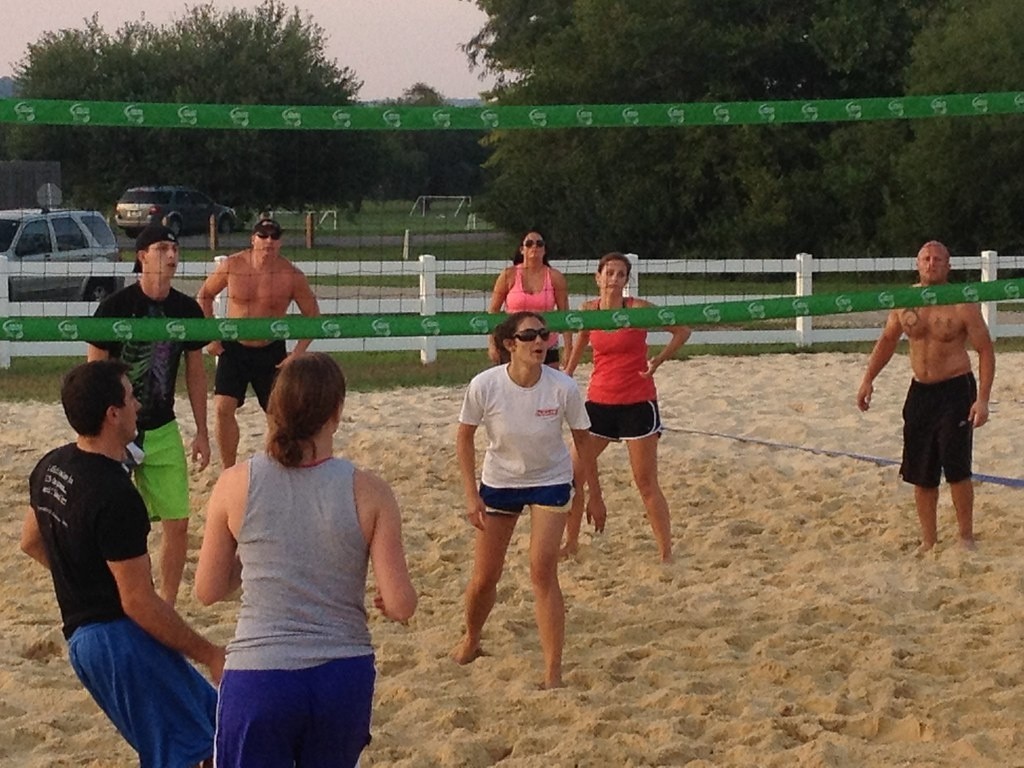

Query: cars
(0, 206), (125, 303)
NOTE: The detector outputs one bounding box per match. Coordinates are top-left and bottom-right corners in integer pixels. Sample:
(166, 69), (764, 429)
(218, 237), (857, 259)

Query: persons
(558, 252), (690, 563)
(194, 353), (420, 768)
(88, 224), (211, 605)
(21, 361), (228, 768)
(486, 229), (573, 377)
(452, 310), (605, 689)
(197, 219), (320, 468)
(857, 240), (995, 555)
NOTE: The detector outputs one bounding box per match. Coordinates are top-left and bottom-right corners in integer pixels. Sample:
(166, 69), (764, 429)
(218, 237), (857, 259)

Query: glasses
(256, 232), (281, 240)
(513, 329), (550, 342)
(522, 239), (546, 248)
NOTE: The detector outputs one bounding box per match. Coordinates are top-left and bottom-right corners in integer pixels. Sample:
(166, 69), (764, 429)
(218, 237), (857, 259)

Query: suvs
(114, 183), (238, 239)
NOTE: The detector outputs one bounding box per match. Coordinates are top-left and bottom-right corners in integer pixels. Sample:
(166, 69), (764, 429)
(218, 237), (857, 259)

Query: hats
(131, 222), (179, 273)
(253, 219), (281, 231)
